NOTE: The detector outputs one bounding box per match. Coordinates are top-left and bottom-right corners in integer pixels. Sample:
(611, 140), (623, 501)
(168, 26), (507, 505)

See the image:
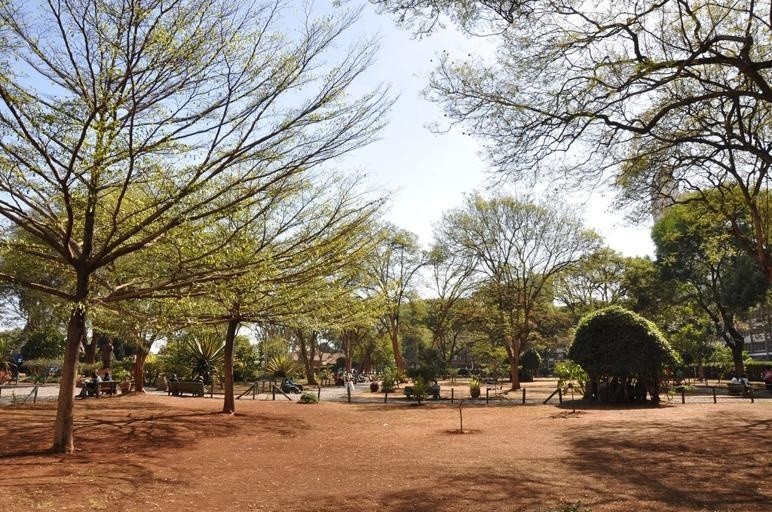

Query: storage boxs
(76, 381), (121, 399)
(168, 381), (204, 397)
(727, 383), (746, 396)
(403, 385), (440, 400)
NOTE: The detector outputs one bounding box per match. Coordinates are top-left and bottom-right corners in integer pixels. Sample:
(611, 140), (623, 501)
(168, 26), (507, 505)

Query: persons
(761, 368), (772, 390)
(282, 377), (296, 393)
(168, 375), (177, 395)
(432, 379), (435, 387)
(319, 368), (358, 386)
(192, 376), (203, 396)
(434, 381), (440, 399)
(732, 373), (750, 395)
(75, 372), (111, 399)
